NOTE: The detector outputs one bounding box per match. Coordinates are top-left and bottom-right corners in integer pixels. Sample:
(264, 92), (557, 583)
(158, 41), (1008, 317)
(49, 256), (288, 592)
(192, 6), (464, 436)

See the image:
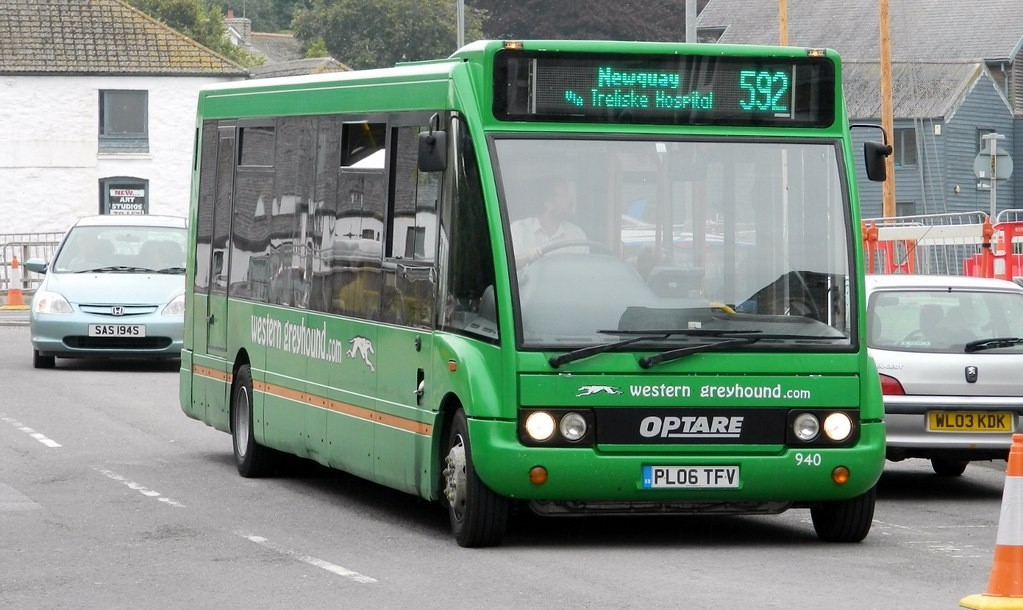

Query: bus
(178, 35), (891, 554)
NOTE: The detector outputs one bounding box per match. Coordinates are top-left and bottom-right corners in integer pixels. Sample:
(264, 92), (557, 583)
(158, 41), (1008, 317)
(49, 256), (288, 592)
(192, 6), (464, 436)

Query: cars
(825, 271), (1023, 483)
(23, 213), (190, 369)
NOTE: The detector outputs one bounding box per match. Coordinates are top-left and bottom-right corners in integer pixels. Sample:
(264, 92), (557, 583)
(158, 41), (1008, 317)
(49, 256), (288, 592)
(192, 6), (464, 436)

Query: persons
(509, 180), (589, 273)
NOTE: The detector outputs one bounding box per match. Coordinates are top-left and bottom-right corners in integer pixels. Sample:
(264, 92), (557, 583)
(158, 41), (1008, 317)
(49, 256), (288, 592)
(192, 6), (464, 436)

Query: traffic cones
(959, 434), (1023, 610)
(0, 254), (32, 310)
